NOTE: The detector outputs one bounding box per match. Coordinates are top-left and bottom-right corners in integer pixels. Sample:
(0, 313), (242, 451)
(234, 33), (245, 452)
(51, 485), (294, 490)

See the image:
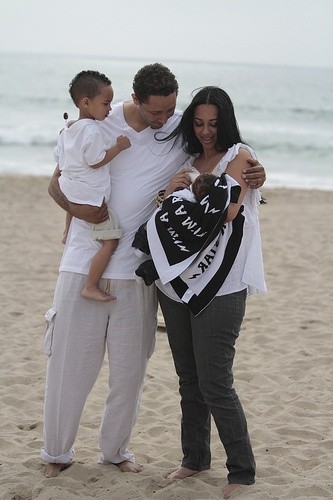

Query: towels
(146, 172), (246, 317)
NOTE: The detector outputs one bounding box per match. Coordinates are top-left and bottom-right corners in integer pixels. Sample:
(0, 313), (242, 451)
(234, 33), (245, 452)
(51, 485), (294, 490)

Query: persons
(53, 69), (132, 302)
(133, 172), (221, 286)
(150, 85), (258, 500)
(38, 62), (266, 479)
